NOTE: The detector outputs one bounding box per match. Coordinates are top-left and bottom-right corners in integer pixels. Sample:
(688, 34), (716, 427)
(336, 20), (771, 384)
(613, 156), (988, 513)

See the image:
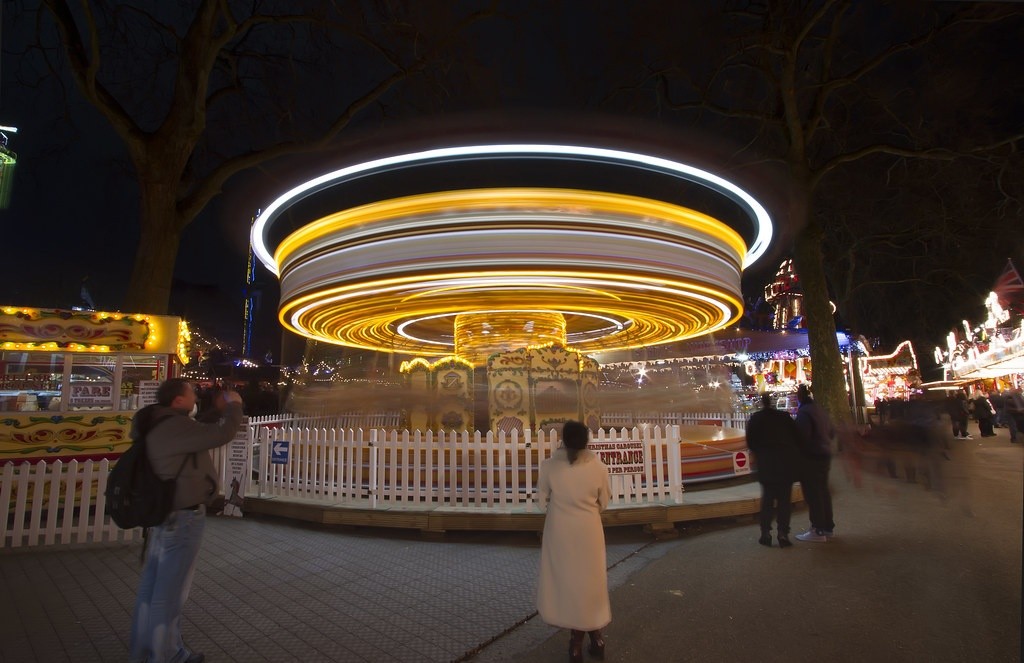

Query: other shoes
(184, 654), (205, 663)
(779, 540), (792, 547)
(759, 539), (771, 547)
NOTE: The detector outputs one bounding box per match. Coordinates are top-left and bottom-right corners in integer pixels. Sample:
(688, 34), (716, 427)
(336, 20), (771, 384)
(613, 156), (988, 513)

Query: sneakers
(796, 528), (826, 542)
(823, 530), (833, 536)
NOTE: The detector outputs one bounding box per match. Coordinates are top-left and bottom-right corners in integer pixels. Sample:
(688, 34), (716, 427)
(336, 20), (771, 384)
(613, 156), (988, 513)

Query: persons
(795, 384), (836, 542)
(1002, 385), (1024, 443)
(538, 420), (612, 663)
(947, 393), (973, 440)
(874, 397), (902, 422)
(128, 377), (242, 663)
(746, 393), (799, 549)
(972, 389), (997, 437)
(989, 389), (1008, 428)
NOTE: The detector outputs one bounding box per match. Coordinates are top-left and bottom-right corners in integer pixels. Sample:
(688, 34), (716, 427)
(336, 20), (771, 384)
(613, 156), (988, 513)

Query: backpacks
(106, 414), (188, 529)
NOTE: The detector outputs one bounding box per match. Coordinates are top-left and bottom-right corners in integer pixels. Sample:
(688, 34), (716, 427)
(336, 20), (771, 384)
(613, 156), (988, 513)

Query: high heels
(588, 640), (605, 659)
(569, 647), (584, 663)
